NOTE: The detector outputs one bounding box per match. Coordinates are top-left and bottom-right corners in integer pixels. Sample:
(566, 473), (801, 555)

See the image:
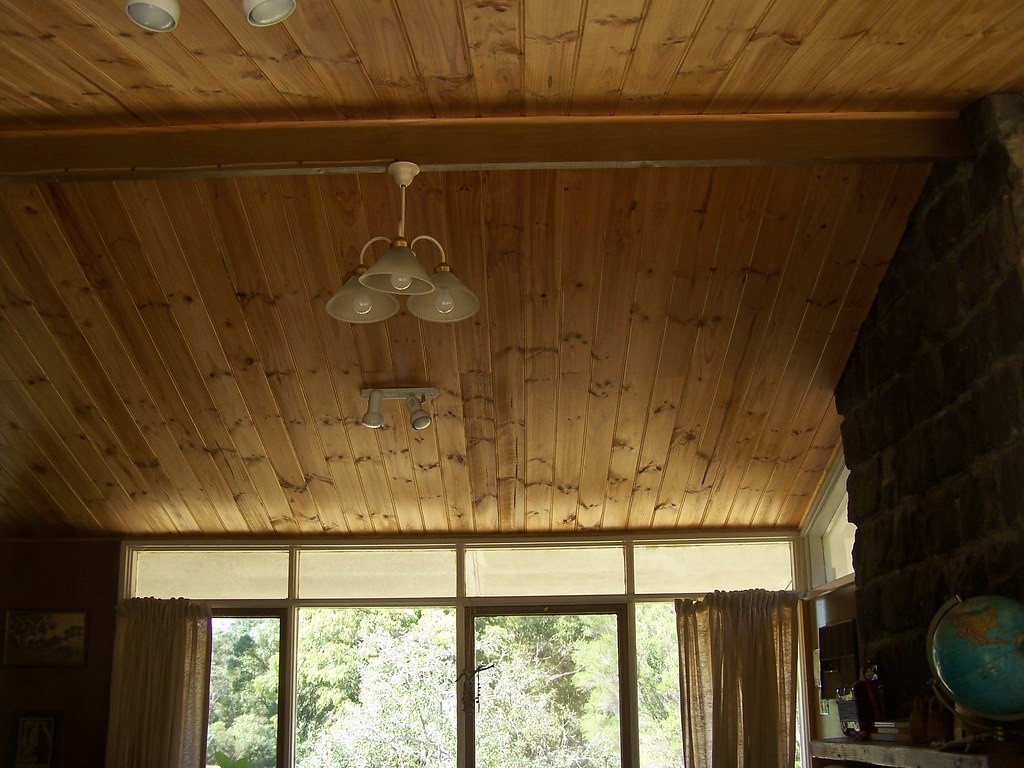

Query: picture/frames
(1, 608), (90, 668)
(7, 709), (61, 768)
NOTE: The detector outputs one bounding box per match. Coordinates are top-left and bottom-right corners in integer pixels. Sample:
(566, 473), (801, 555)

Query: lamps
(325, 161), (482, 324)
(125, 0), (181, 33)
(242, 0), (298, 27)
(356, 388), (439, 431)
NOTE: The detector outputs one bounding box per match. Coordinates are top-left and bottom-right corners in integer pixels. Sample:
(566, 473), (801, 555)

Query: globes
(926, 591), (1024, 756)
